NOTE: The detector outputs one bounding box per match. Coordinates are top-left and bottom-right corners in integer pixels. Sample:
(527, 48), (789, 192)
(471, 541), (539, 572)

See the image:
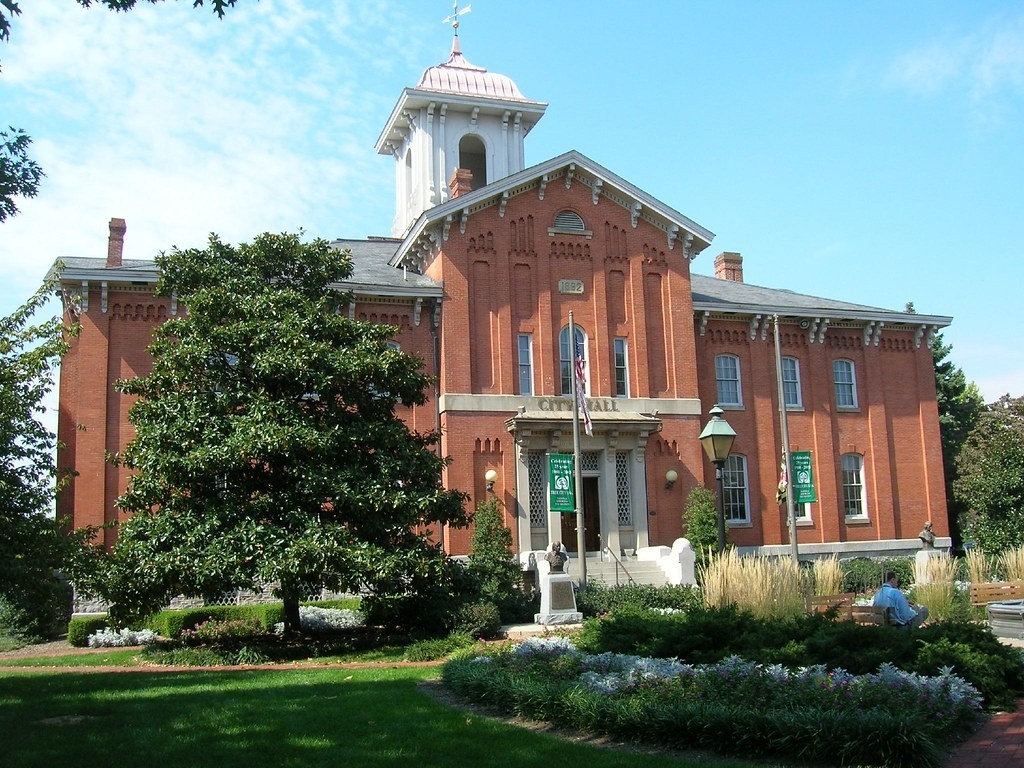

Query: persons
(873, 571), (929, 629)
(544, 541), (567, 571)
(918, 521), (936, 551)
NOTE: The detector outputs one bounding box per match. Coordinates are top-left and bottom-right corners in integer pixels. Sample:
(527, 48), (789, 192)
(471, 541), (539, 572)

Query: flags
(547, 452), (576, 513)
(776, 444), (786, 506)
(574, 328), (594, 437)
(788, 452), (815, 504)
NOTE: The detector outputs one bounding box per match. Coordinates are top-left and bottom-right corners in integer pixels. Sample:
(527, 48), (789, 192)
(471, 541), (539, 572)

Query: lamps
(485, 470), (499, 491)
(665, 470), (677, 489)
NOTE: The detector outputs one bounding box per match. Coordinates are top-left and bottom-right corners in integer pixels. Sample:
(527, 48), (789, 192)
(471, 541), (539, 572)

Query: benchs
(816, 605), (913, 629)
(806, 590), (855, 616)
(970, 581), (1024, 623)
